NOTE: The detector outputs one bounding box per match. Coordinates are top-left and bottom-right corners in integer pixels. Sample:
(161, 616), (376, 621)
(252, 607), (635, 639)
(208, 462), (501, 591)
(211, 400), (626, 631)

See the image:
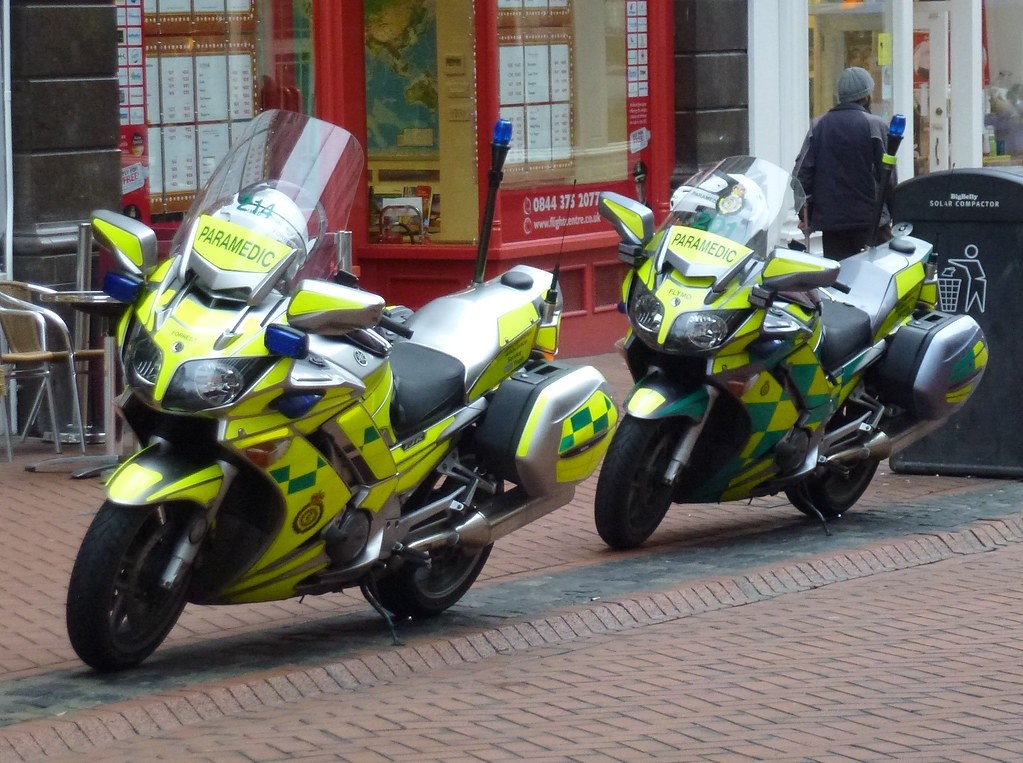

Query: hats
(838, 66), (874, 103)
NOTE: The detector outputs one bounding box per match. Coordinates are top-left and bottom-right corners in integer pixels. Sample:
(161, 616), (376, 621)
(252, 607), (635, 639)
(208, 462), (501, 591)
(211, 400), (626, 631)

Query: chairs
(0, 280), (106, 462)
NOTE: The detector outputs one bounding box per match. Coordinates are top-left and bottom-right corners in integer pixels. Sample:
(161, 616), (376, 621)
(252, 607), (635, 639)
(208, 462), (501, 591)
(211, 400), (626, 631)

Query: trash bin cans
(885, 163), (1023, 479)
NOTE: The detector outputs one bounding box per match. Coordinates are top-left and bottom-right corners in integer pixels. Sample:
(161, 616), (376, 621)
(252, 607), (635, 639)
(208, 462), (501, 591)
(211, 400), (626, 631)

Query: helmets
(669, 168), (767, 245)
(211, 183), (318, 285)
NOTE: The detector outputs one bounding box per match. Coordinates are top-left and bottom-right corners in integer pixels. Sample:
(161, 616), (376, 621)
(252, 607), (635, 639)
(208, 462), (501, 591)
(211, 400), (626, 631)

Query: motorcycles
(66, 110), (620, 672)
(593, 114), (992, 552)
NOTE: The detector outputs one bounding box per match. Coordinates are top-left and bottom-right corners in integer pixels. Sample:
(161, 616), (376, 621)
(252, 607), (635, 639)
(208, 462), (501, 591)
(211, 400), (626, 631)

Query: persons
(791, 67), (899, 261)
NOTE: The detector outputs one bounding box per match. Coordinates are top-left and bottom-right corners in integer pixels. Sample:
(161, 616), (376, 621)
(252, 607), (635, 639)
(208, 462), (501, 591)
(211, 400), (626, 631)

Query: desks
(23, 290), (133, 481)
